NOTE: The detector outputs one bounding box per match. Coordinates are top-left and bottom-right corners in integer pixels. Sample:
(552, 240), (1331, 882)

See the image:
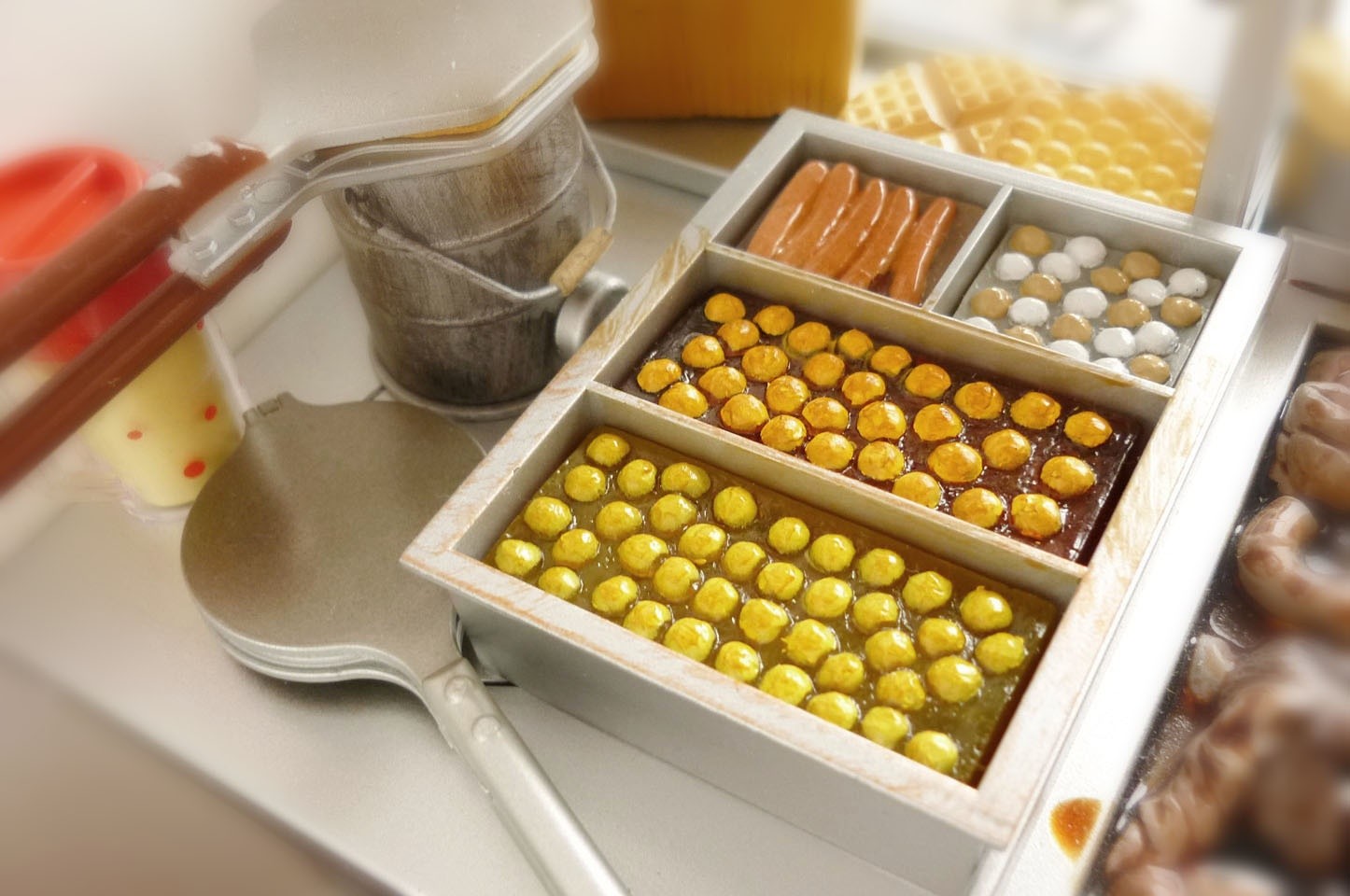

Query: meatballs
(965, 78), (1242, 391)
(502, 293), (1131, 774)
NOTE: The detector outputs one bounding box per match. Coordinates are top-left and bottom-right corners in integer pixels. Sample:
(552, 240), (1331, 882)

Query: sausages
(751, 154), (958, 308)
(1107, 339), (1349, 896)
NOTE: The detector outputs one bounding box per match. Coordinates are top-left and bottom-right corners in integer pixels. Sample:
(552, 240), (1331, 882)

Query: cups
(2, 146), (254, 511)
